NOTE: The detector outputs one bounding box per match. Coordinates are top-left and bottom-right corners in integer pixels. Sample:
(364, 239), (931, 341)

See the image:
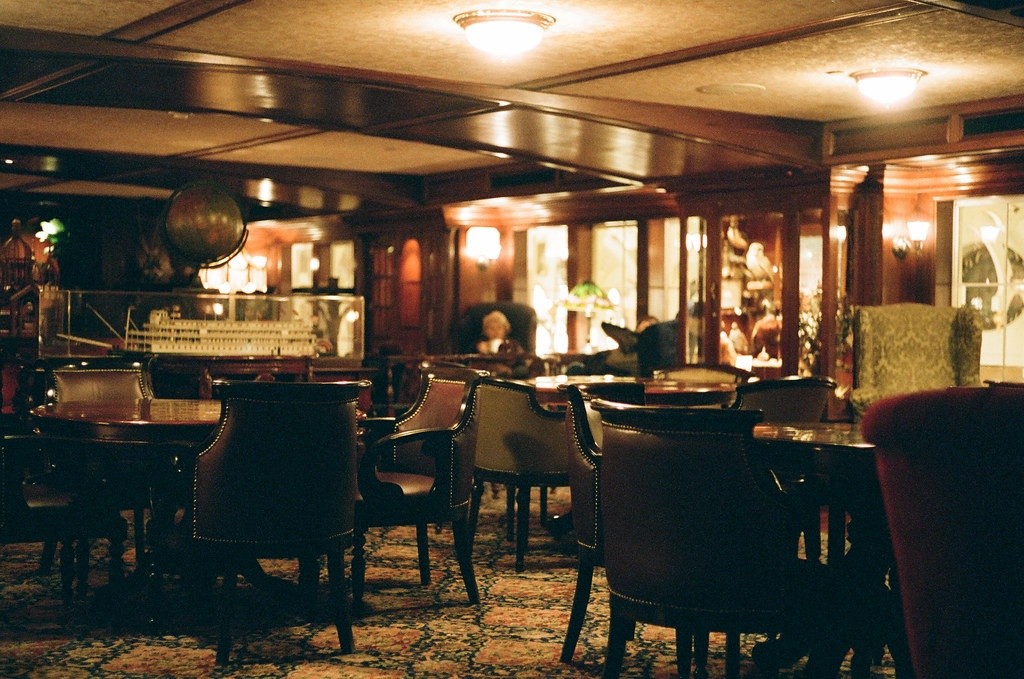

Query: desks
(31, 400), (367, 598)
(753, 423), (877, 566)
(496, 373), (738, 405)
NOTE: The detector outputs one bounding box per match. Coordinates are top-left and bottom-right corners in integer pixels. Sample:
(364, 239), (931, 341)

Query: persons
(467, 311), (525, 354)
(14, 300), (36, 329)
(607, 303), (704, 372)
(716, 303), (789, 375)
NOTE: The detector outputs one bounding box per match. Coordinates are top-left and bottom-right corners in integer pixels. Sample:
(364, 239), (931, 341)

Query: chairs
(1, 301), (1024, 679)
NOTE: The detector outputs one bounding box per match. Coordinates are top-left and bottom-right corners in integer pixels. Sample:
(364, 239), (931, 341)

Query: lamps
(560, 280), (613, 353)
(453, 7), (556, 59)
(464, 227), (503, 273)
(852, 68), (927, 108)
(892, 221), (932, 260)
